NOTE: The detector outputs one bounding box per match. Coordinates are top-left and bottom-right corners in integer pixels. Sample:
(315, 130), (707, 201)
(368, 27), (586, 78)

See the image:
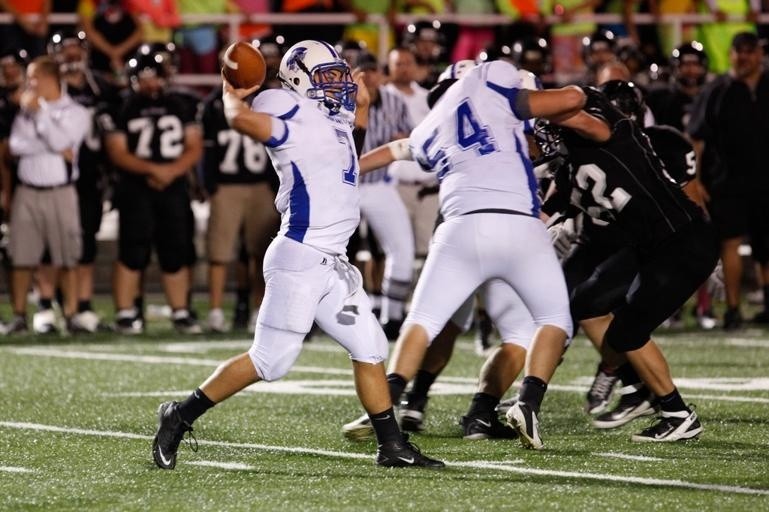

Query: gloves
(547, 225), (579, 258)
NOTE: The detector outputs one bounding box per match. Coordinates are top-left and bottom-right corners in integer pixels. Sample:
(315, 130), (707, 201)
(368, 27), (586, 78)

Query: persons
(153, 42), (445, 469)
(530, 77), (719, 443)
(0, 0), (769, 334)
(344, 59), (586, 452)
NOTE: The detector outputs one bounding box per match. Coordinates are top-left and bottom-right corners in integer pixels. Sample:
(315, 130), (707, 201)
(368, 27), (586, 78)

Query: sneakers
(154, 399), (189, 472)
(341, 393), (426, 439)
(376, 436), (446, 470)
(584, 360), (704, 444)
(457, 393), (544, 451)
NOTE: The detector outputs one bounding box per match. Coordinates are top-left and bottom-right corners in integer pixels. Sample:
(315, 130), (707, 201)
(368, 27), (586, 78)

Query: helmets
(125, 43), (178, 93)
(404, 19), (445, 63)
(50, 33), (90, 77)
(533, 79), (643, 158)
(276, 38), (358, 118)
(579, 29), (709, 89)
(436, 60), (481, 81)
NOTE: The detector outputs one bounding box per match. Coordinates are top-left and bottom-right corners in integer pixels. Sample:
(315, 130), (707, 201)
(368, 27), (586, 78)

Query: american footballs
(224, 41), (266, 91)
